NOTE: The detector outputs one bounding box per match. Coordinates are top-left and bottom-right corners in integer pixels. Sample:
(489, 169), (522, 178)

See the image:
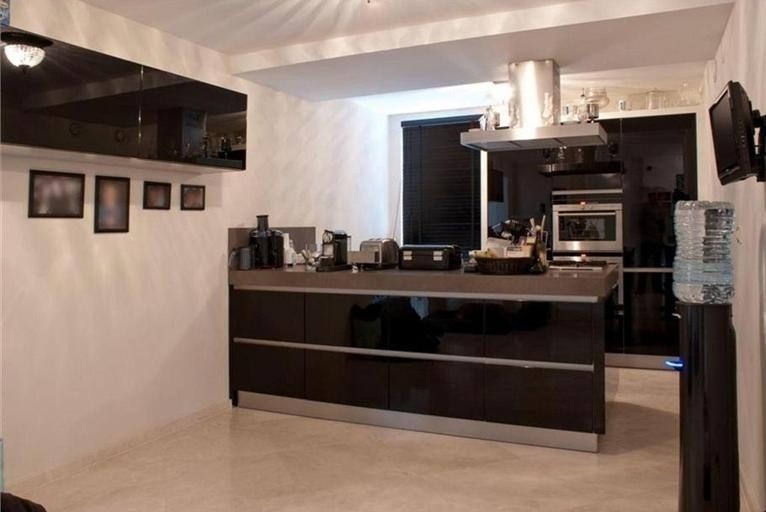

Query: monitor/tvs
(709, 80), (766, 185)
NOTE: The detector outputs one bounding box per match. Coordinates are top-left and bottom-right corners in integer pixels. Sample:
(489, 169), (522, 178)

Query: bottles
(671, 200), (736, 304)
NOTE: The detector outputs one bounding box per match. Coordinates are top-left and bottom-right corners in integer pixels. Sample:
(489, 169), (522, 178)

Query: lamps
(0, 31), (52, 74)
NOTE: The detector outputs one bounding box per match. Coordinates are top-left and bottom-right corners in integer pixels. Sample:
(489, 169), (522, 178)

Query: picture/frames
(181, 184), (205, 211)
(142, 181), (171, 210)
(27, 169), (85, 218)
(94, 176), (130, 233)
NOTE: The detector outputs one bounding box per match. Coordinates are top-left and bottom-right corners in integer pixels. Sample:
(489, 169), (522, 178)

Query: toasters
(348, 237), (399, 270)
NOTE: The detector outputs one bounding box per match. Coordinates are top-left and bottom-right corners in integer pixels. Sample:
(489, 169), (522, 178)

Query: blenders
(248, 213), (285, 269)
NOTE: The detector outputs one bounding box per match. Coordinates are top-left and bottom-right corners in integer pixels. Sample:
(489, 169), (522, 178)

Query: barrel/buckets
(672, 200), (737, 303)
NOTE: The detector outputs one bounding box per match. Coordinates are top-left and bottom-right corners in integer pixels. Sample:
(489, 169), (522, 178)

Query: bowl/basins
(475, 257), (535, 275)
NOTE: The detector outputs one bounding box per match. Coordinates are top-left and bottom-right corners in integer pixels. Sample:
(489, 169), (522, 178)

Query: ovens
(550, 188), (624, 306)
